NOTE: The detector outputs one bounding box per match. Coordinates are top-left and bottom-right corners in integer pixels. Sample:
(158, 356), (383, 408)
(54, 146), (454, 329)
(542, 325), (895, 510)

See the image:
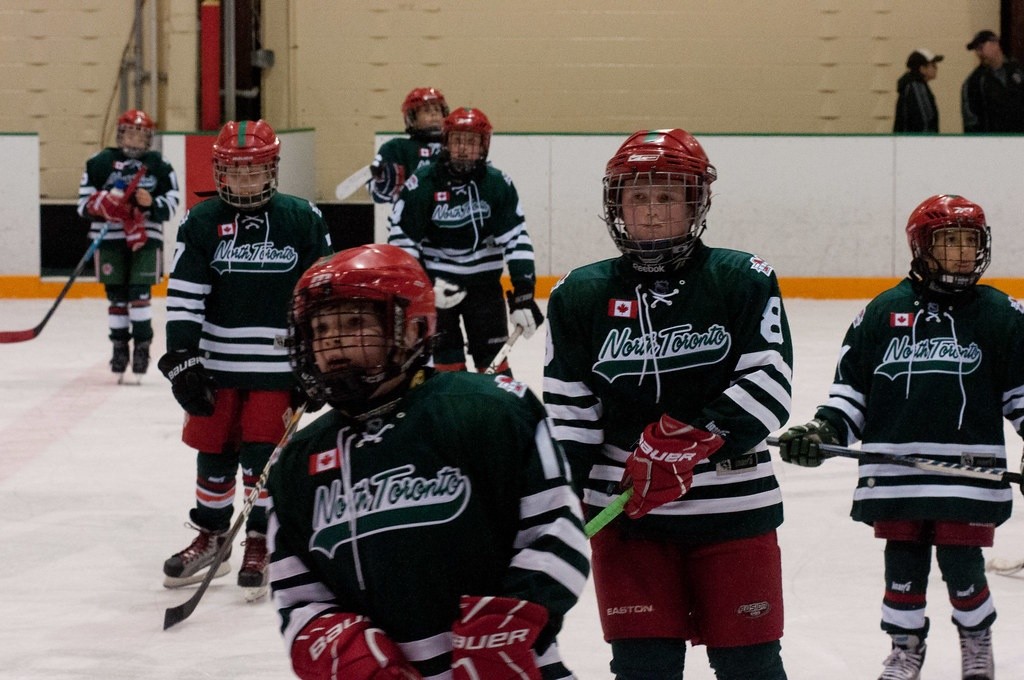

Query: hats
(967, 30), (1000, 50)
(907, 49), (944, 69)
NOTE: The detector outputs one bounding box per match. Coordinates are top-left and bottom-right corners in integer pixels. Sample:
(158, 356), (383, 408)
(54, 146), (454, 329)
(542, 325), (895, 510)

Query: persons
(778, 197), (1024, 680)
(540, 130), (792, 680)
(958, 29), (1024, 135)
(266, 243), (589, 680)
(365, 88), (451, 204)
(892, 48), (945, 135)
(390, 108), (545, 378)
(77, 110), (178, 376)
(157, 118), (330, 589)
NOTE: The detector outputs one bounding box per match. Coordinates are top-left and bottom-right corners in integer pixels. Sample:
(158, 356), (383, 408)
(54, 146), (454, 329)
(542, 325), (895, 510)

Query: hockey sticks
(583, 486), (635, 539)
(484, 324), (524, 375)
(765, 435), (1021, 483)
(162, 400), (308, 629)
(0, 165), (148, 343)
(336, 160), (379, 201)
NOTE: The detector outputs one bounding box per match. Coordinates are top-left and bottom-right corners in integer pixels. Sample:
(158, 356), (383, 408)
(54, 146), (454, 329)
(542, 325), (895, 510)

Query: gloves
(426, 272), (468, 310)
(86, 190), (147, 252)
(291, 612), (421, 680)
(778, 418), (838, 467)
(157, 350), (219, 416)
(620, 416), (724, 519)
(506, 278), (544, 340)
(287, 362), (328, 414)
(451, 593), (548, 680)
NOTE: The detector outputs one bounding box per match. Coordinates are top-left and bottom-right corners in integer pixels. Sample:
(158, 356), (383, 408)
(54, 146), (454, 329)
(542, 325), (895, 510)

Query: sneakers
(162, 521), (232, 588)
(876, 633), (927, 680)
(131, 339), (152, 385)
(237, 534), (270, 602)
(109, 339), (130, 384)
(957, 625), (994, 680)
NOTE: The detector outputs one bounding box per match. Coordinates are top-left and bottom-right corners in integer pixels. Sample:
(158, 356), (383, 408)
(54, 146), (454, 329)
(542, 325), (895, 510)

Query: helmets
(603, 128), (718, 220)
(441, 107), (492, 149)
(292, 243), (436, 365)
(906, 194), (988, 260)
(212, 119), (280, 166)
(115, 109), (155, 135)
(402, 87), (449, 128)
